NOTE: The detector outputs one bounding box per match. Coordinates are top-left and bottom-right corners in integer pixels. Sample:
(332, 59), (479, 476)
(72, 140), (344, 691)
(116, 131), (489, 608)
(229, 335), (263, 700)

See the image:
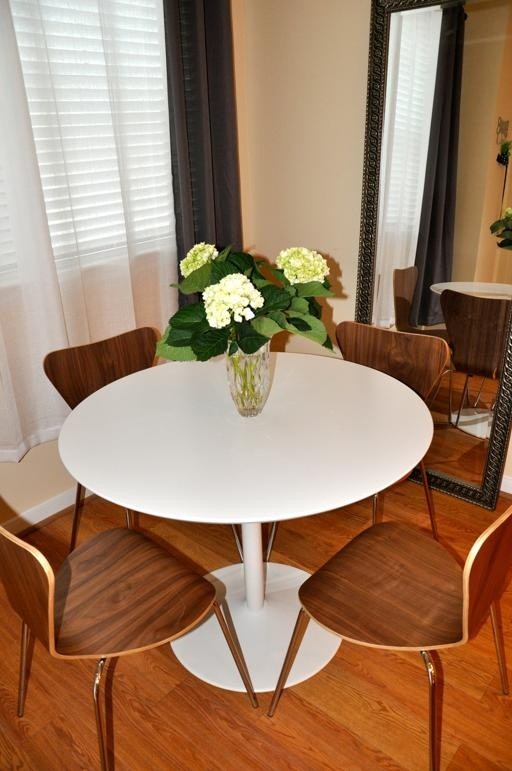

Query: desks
(58, 352), (434, 694)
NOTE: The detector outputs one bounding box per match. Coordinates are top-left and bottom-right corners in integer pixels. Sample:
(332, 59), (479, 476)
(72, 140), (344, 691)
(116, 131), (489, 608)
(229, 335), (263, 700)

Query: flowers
(488, 206), (512, 252)
(154, 238), (338, 399)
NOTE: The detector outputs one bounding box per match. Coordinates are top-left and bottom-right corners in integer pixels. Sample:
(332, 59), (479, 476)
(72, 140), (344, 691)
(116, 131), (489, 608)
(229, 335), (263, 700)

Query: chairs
(335, 322), (449, 543)
(1, 525), (259, 771)
(268, 506), (511, 771)
(43, 328), (160, 552)
(391, 264), (512, 440)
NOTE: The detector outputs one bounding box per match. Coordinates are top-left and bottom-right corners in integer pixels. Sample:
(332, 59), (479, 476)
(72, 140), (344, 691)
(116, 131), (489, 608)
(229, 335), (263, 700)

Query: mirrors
(349, 0), (511, 514)
